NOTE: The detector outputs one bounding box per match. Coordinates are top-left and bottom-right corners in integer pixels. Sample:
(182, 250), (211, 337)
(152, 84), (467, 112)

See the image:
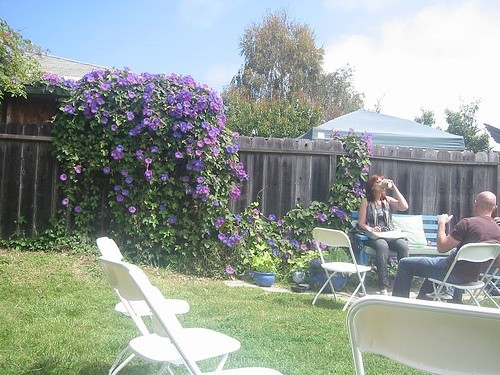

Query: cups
(381, 179), (392, 189)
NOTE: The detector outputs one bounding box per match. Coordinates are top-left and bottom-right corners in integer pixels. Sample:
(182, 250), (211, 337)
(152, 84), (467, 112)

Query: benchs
(349, 210), (457, 291)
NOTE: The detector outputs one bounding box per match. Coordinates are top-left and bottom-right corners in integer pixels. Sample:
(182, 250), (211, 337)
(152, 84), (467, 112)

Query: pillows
(390, 214), (427, 246)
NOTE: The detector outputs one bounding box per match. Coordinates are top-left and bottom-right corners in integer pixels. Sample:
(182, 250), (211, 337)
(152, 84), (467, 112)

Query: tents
(295, 110), (465, 152)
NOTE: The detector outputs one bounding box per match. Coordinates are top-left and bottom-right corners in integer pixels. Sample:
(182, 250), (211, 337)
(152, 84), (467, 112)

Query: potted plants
(251, 251), (283, 287)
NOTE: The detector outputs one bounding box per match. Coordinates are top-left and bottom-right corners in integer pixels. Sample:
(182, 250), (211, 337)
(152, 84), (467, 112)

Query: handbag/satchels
(365, 229), (407, 240)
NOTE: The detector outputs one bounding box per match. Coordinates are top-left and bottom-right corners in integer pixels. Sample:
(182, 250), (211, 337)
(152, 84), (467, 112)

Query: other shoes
(380, 289), (388, 296)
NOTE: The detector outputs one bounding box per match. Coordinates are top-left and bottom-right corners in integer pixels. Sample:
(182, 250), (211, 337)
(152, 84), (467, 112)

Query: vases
(304, 265), (353, 294)
(19, 84), (74, 97)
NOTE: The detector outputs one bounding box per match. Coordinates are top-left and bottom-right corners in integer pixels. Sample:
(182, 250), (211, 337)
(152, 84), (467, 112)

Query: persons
(493, 217), (500, 225)
(391, 191), (500, 303)
(358, 175), (410, 295)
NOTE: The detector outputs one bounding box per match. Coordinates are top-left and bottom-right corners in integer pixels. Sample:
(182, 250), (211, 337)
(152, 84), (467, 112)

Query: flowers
(282, 240), (354, 284)
(21, 71), (74, 95)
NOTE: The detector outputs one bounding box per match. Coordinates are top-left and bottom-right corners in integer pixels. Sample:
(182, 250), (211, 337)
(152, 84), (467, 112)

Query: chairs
(94, 256), (242, 375)
(346, 294), (500, 375)
(96, 236), (190, 375)
(311, 227), (373, 312)
(428, 243), (500, 310)
(109, 260), (285, 375)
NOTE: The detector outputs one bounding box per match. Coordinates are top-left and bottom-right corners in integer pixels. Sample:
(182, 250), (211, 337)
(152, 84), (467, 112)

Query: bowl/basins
(291, 286), (307, 293)
